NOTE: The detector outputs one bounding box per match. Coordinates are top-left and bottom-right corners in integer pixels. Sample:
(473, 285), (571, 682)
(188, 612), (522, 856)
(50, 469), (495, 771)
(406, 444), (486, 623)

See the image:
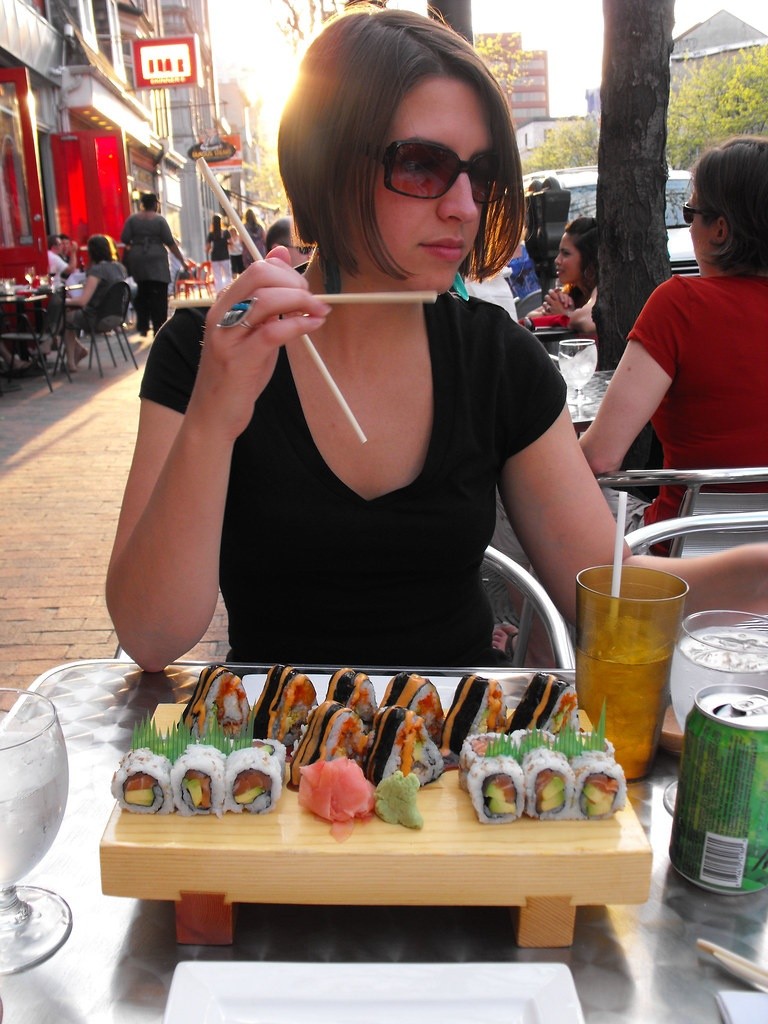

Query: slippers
(14, 363), (33, 373)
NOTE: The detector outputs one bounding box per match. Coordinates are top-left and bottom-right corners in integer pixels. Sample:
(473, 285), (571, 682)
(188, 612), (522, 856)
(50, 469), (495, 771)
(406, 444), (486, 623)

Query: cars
(514, 164), (703, 280)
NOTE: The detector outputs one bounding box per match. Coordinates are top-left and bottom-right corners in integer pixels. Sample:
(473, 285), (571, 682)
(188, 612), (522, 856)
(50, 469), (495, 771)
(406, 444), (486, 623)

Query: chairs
(595, 467), (768, 558)
(52, 280), (140, 380)
(515, 286), (573, 358)
(114, 547), (580, 671)
(0, 284), (72, 393)
(176, 262), (219, 301)
(623, 510), (768, 556)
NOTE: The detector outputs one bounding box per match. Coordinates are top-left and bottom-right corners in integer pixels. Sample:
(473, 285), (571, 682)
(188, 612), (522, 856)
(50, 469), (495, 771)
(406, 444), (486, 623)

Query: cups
(4, 278), (15, 295)
(574, 565), (688, 780)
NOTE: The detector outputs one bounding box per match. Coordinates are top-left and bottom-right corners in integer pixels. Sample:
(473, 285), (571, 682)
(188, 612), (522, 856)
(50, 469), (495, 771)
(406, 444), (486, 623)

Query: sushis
(109, 664), (627, 825)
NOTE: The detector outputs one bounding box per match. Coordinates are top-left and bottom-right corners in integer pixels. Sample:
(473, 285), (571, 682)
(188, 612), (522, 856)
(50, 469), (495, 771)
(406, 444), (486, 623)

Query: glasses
(284, 245), (317, 254)
(361, 140), (510, 204)
(682, 201), (719, 223)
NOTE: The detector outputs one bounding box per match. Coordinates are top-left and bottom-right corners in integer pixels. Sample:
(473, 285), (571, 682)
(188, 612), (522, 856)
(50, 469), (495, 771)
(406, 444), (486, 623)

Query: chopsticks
(169, 154), (436, 445)
(696, 938), (768, 985)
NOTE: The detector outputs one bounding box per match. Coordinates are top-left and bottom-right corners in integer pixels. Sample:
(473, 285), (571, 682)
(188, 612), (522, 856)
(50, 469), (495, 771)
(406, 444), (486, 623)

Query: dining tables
(0, 658), (768, 1024)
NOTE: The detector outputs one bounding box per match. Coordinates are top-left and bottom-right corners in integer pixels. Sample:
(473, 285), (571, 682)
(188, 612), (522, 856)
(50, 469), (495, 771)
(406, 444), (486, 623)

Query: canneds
(669, 683), (768, 895)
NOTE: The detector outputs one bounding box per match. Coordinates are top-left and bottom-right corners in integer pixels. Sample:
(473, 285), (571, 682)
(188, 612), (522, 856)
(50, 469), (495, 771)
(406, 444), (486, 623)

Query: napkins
(715, 990), (768, 1024)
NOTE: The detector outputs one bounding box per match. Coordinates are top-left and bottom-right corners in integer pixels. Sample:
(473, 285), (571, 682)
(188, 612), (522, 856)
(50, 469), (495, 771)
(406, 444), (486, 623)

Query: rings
(215, 297), (258, 329)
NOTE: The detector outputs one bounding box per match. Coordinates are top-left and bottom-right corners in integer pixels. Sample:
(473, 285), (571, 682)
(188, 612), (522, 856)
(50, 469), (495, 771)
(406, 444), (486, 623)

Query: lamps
(63, 24), (75, 48)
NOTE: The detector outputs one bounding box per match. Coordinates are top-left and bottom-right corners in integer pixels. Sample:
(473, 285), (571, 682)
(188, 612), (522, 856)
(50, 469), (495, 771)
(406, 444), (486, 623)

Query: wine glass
(557, 339), (599, 422)
(24, 266), (35, 290)
(665, 610), (768, 821)
(47, 266), (59, 289)
(1, 688), (73, 976)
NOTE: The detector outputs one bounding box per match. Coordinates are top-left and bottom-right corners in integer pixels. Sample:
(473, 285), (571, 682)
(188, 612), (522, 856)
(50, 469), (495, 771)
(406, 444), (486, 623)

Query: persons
(518, 177), (561, 242)
(526, 217), (597, 332)
(240, 210), (266, 267)
(120, 194), (194, 337)
(266, 216), (315, 266)
(59, 234), (70, 262)
(492, 137), (768, 653)
(27, 234), (126, 372)
(108, 9), (768, 676)
(228, 226), (244, 278)
(0, 339), (31, 370)
(205, 214), (232, 291)
(46, 235), (77, 278)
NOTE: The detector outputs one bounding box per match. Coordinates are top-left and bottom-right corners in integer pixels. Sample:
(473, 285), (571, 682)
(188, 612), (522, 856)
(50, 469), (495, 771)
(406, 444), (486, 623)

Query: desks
(0, 282), (85, 382)
(560, 369), (618, 439)
